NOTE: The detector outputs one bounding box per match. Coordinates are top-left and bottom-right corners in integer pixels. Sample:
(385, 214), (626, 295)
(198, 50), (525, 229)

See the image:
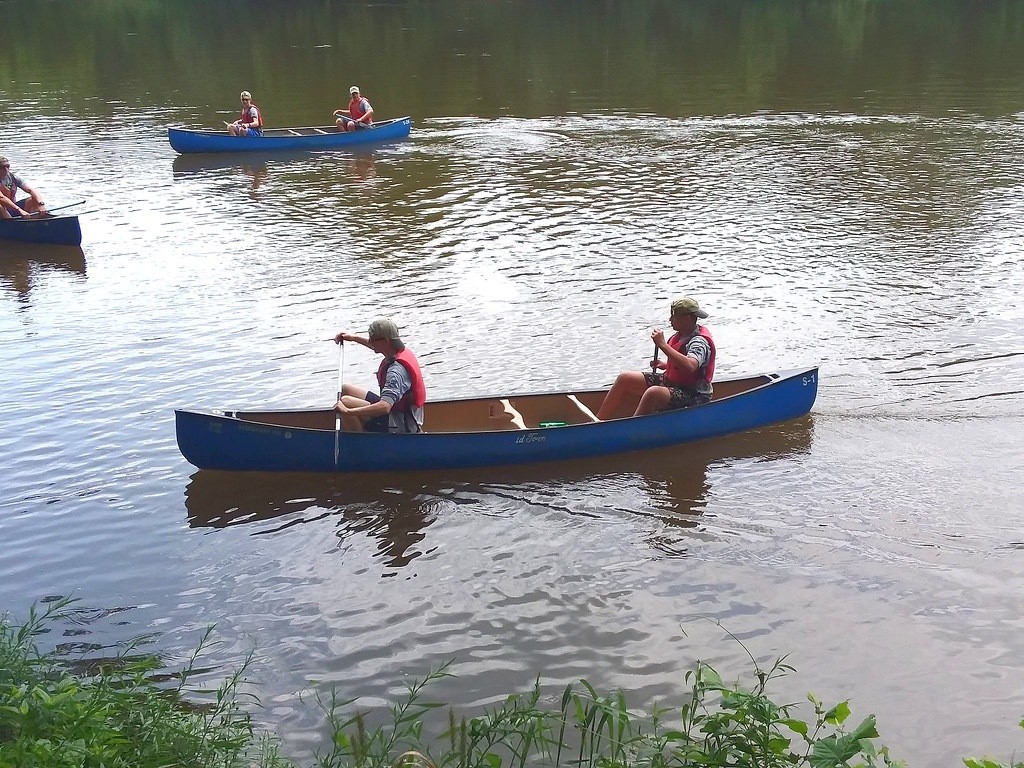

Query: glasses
(242, 98), (251, 101)
(671, 310), (691, 316)
(369, 336), (386, 342)
(0, 164), (10, 168)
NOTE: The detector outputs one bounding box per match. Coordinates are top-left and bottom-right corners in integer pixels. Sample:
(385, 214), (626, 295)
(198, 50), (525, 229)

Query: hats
(349, 85), (360, 94)
(0, 156), (8, 162)
(368, 319), (404, 348)
(671, 296), (709, 319)
(241, 90), (253, 101)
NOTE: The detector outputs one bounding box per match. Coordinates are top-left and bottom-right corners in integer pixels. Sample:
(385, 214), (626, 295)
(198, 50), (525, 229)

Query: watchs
(38, 202), (45, 205)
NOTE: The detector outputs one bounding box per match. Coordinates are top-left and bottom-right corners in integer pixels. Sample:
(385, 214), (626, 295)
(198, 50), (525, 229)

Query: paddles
(335, 113), (377, 131)
(0, 200), (87, 221)
(223, 121), (247, 129)
(651, 343), (661, 376)
(333, 338), (344, 468)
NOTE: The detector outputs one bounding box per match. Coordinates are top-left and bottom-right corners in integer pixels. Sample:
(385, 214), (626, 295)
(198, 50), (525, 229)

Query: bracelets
(249, 123), (250, 127)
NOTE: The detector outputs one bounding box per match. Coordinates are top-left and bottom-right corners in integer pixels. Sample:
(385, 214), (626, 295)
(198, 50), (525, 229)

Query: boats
(174, 365), (821, 469)
(0, 209), (81, 246)
(168, 116), (411, 154)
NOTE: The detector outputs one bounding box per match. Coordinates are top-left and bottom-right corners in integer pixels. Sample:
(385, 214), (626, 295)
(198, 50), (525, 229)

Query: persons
(226, 91), (264, 137)
(334, 85), (374, 132)
(0, 156), (46, 219)
(333, 319), (426, 432)
(595, 298), (716, 420)
